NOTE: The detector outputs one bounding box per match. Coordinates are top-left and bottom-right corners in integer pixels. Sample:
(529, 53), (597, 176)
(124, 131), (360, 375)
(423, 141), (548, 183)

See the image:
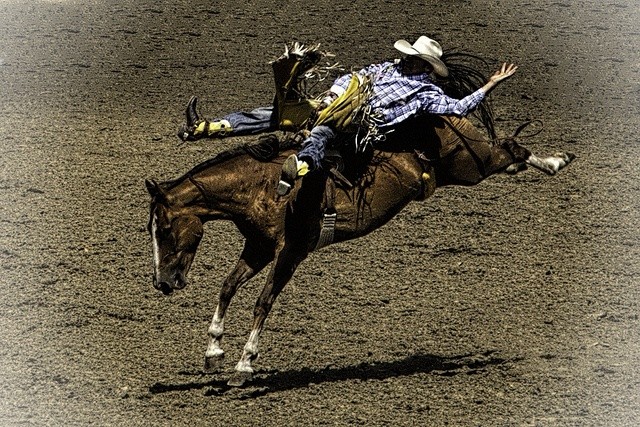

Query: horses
(143, 45), (577, 388)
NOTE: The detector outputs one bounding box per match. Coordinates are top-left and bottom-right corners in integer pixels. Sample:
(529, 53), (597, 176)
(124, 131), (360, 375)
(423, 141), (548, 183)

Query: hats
(394, 34), (450, 77)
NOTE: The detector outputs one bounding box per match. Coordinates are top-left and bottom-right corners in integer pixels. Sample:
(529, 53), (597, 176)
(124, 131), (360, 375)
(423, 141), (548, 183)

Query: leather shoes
(276, 153), (298, 195)
(178, 95), (200, 141)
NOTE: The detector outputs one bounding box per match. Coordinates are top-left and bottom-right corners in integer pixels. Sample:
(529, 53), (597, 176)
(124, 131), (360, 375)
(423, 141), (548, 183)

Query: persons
(177, 34), (519, 196)
